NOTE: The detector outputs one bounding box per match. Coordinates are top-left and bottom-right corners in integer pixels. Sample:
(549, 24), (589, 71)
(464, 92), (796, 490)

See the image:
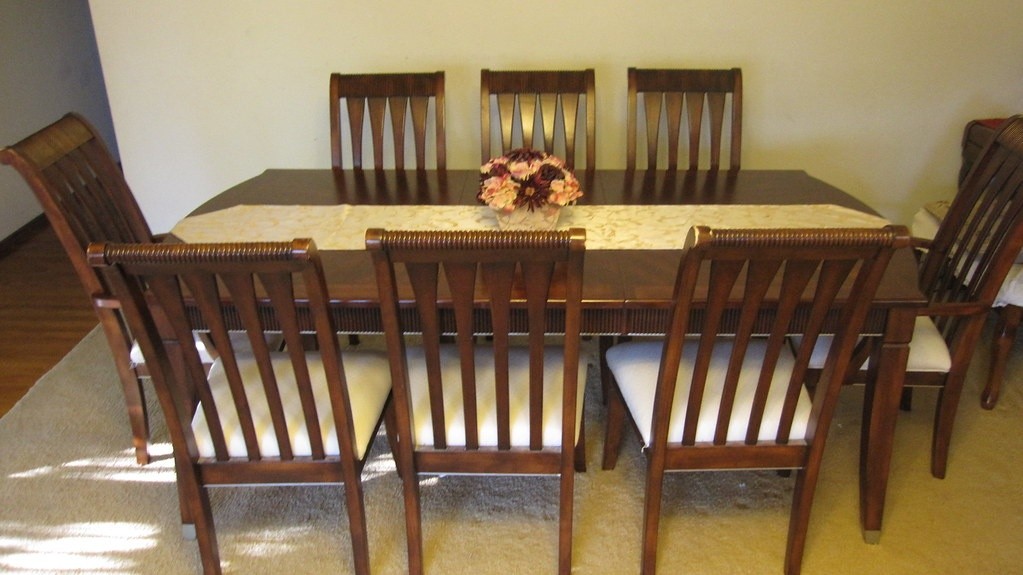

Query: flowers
(478, 149), (584, 211)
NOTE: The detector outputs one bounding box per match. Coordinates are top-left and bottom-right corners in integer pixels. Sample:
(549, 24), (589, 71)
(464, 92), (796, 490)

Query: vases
(497, 200), (560, 231)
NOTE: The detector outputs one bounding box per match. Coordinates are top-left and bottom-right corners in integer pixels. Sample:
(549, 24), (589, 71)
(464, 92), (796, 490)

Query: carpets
(0, 307), (1023, 575)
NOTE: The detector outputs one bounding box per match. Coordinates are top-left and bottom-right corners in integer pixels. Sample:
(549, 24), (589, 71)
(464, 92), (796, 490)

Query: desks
(151, 168), (931, 541)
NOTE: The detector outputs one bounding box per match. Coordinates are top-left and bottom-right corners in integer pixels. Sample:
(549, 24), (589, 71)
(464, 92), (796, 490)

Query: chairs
(0, 67), (1023, 575)
